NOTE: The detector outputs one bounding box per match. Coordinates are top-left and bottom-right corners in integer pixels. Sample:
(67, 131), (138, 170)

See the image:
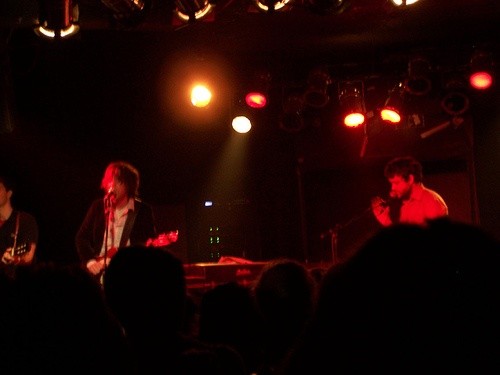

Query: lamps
(182, 44), (495, 134)
(31, 0), (422, 42)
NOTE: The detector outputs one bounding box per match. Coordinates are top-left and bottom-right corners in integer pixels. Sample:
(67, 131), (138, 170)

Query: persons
(372, 154), (449, 227)
(0, 172), (41, 273)
(0, 217), (500, 375)
(77, 161), (158, 279)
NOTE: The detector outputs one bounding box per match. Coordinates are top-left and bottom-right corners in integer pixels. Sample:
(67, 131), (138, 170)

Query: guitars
(85, 228), (181, 290)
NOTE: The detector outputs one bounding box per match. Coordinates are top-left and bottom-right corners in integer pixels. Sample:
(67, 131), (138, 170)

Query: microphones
(106, 187), (116, 199)
(380, 190), (398, 214)
